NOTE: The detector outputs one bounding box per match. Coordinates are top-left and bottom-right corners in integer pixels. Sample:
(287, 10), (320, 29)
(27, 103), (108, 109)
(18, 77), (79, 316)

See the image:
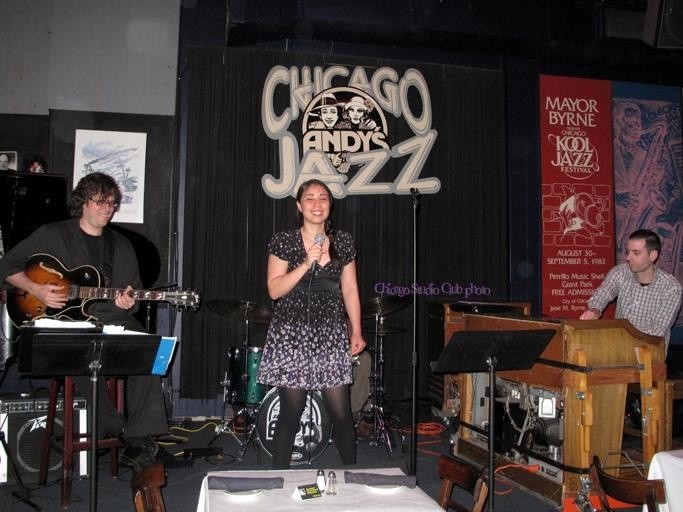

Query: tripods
(207, 325), (408, 470)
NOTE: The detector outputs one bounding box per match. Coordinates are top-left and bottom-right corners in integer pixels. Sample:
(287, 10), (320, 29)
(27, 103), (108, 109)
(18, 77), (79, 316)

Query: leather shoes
(118, 436), (190, 468)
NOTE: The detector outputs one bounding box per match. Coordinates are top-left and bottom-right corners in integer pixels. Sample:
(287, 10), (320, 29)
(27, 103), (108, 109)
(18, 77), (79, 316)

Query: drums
(254, 386), (333, 465)
(220, 344), (272, 408)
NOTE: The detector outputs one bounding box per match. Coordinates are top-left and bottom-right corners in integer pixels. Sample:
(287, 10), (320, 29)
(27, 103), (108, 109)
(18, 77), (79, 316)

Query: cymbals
(361, 326), (406, 333)
(345, 293), (414, 320)
(206, 297), (274, 324)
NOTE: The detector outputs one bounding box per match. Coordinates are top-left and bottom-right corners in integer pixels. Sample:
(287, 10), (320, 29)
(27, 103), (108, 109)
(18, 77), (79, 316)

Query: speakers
(0, 398), (87, 487)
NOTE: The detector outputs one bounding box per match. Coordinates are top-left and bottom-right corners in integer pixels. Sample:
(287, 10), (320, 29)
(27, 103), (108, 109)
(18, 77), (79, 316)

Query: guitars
(6, 254), (201, 328)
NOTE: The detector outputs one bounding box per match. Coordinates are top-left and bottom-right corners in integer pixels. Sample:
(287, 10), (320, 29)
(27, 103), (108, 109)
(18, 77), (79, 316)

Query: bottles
(317, 469), (337, 496)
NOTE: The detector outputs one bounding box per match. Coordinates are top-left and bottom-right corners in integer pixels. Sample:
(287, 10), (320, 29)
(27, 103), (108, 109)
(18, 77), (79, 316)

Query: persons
(307, 92), (347, 131)
(0, 171), (169, 467)
(579, 228), (683, 421)
(335, 97), (375, 129)
(253, 178), (367, 467)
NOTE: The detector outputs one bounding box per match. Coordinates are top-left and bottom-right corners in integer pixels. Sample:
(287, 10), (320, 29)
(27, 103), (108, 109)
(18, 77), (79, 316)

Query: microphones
(309, 233), (325, 274)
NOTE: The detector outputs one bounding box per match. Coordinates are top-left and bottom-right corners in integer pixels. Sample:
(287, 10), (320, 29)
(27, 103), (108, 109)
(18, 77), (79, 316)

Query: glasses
(89, 198), (118, 207)
